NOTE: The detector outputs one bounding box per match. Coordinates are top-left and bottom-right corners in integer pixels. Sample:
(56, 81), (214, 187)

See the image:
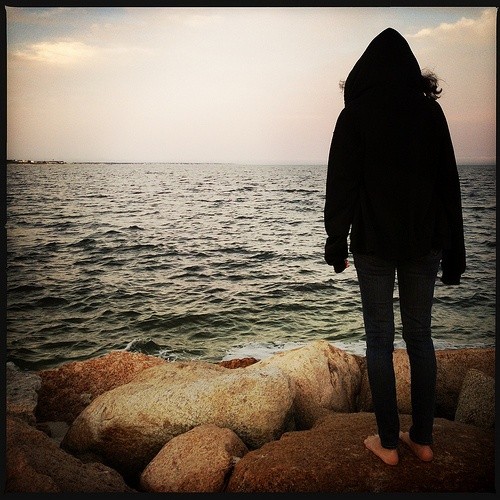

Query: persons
(323, 27), (465, 466)
